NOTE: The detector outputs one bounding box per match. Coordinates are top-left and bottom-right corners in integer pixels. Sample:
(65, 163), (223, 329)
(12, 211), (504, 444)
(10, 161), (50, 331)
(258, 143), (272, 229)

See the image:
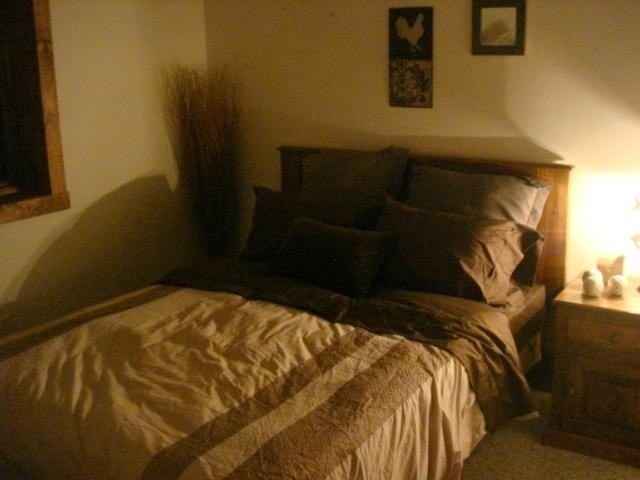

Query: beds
(0, 144), (573, 479)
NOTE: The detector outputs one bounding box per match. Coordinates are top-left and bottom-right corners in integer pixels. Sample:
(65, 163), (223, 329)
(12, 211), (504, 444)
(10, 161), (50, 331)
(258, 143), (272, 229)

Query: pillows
(280, 146), (409, 199)
(269, 217), (395, 296)
(374, 199), (545, 306)
(243, 186), (384, 275)
(402, 158), (551, 230)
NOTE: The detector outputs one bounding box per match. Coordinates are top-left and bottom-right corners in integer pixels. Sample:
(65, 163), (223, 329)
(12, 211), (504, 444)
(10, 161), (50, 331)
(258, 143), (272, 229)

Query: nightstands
(540, 270), (640, 469)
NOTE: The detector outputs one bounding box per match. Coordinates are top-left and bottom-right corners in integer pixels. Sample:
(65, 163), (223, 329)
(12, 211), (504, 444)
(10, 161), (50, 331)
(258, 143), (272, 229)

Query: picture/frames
(471, 0), (524, 56)
